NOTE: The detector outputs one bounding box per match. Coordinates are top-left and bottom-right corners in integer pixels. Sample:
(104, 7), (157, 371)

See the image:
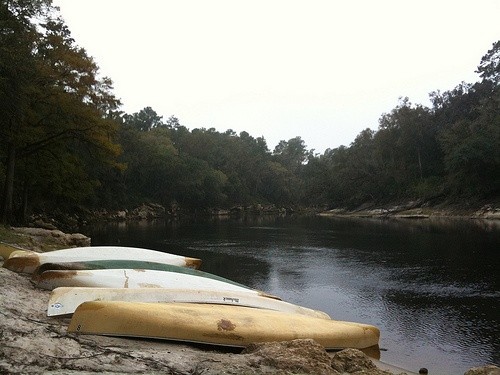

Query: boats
(47, 286), (331, 320)
(66, 299), (381, 350)
(0, 238), (205, 283)
(29, 263), (257, 293)
